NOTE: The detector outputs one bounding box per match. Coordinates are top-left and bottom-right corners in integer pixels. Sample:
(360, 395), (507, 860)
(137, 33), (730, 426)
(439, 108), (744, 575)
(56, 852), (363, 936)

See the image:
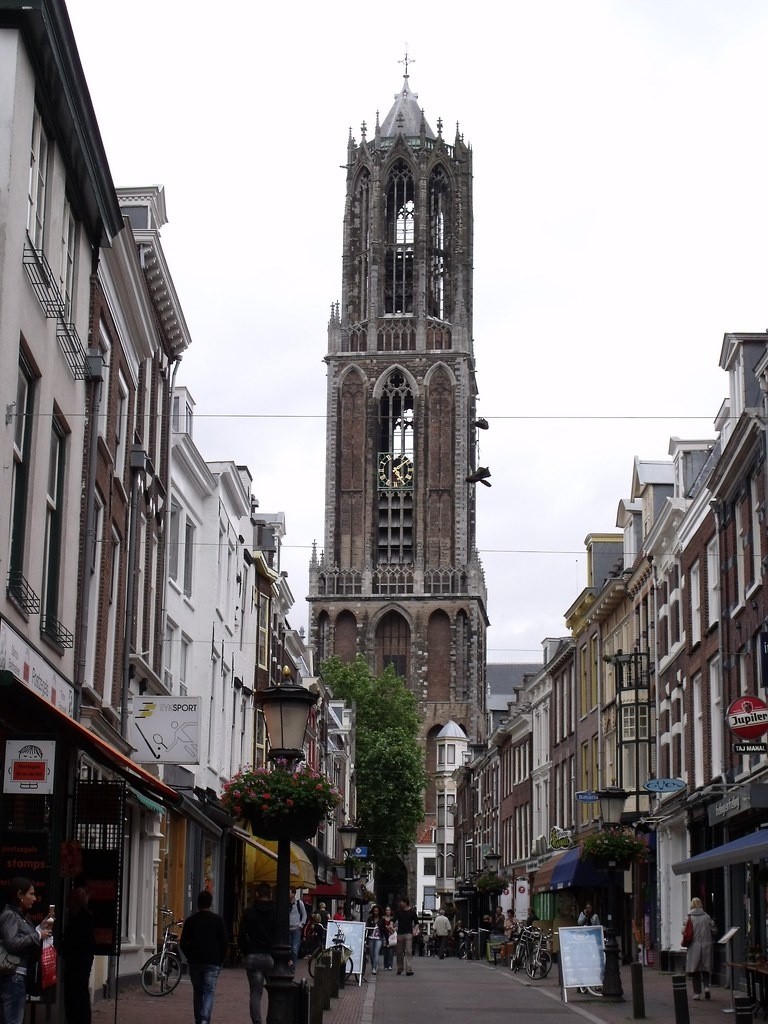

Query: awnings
(309, 873), (346, 896)
(254, 837), (317, 889)
(349, 881), (364, 899)
(533, 844), (610, 892)
(671, 828), (768, 877)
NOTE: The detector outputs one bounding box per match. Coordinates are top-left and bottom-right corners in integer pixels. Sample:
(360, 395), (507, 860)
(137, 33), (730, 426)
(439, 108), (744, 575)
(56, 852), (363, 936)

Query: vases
(596, 852), (618, 868)
(248, 805), (320, 841)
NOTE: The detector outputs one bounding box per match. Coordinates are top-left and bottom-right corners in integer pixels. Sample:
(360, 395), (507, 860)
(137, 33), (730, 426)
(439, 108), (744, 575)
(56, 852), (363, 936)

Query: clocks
(378, 453), (413, 487)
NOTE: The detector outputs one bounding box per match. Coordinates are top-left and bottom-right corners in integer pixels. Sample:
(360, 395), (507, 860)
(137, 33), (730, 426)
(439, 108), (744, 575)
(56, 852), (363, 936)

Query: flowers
(219, 756), (344, 835)
(577, 825), (654, 868)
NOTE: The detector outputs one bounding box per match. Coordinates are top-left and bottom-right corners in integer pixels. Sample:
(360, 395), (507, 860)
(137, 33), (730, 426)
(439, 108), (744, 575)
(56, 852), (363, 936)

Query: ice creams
(49, 904), (55, 918)
(47, 917), (54, 929)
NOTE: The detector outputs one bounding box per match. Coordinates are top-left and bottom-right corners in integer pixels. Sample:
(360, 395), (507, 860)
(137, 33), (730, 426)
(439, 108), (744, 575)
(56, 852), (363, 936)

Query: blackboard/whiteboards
(326, 919), (366, 974)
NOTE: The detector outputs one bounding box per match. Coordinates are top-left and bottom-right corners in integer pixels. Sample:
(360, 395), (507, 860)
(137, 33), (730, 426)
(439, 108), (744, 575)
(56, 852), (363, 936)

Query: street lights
(358, 873), (370, 923)
(430, 907), (439, 955)
(482, 846), (504, 966)
(335, 817), (362, 922)
(594, 778), (625, 1004)
(251, 663), (323, 1024)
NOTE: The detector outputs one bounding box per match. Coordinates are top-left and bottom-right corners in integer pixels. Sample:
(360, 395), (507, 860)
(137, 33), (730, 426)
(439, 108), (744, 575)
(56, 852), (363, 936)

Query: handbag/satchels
(387, 929), (398, 948)
(0, 939), (21, 977)
(681, 913), (694, 947)
(39, 933), (59, 992)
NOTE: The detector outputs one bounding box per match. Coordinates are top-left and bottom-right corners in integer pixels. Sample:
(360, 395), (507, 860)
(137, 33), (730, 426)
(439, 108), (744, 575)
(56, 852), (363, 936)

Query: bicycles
(456, 928), (475, 960)
(505, 920), (559, 980)
(141, 906), (184, 997)
(308, 920), (379, 985)
(578, 938), (610, 996)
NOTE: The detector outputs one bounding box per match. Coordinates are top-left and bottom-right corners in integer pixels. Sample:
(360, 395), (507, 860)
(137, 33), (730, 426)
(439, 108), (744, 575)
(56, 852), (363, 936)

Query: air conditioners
(85, 354), (106, 383)
(130, 447), (148, 470)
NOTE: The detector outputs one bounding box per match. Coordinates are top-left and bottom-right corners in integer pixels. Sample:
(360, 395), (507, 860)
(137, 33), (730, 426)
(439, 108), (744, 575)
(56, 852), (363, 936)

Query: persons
(237, 881), (276, 1024)
(364, 905), (389, 975)
(179, 890), (229, 1024)
(681, 898), (717, 1000)
(527, 907), (539, 927)
(503, 909), (516, 965)
(389, 897), (419, 976)
(454, 919), (466, 958)
(308, 902), (346, 965)
(381, 905), (398, 971)
(289, 885), (307, 974)
(0, 878), (96, 1024)
(577, 901), (601, 992)
(479, 905), (504, 960)
(432, 909), (452, 959)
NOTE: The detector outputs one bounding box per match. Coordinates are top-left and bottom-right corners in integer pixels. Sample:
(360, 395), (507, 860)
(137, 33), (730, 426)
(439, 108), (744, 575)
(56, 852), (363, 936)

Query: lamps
(687, 791), (743, 802)
(633, 821), (661, 828)
(700, 783), (746, 796)
(640, 815), (666, 824)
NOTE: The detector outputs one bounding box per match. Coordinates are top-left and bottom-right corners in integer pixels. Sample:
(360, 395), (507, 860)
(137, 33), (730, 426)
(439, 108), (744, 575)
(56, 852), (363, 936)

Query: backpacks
(313, 922), (324, 942)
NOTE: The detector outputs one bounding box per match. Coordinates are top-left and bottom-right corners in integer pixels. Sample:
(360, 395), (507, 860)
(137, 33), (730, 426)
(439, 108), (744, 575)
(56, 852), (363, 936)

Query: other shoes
(371, 968), (377, 975)
(704, 987), (711, 1000)
(383, 968), (388, 971)
(396, 968), (404, 975)
(389, 966), (392, 970)
(692, 994), (700, 1001)
(406, 972), (413, 976)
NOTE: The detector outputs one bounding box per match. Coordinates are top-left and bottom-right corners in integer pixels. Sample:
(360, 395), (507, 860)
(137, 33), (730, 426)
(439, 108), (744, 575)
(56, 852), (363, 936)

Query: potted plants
(476, 872), (509, 896)
